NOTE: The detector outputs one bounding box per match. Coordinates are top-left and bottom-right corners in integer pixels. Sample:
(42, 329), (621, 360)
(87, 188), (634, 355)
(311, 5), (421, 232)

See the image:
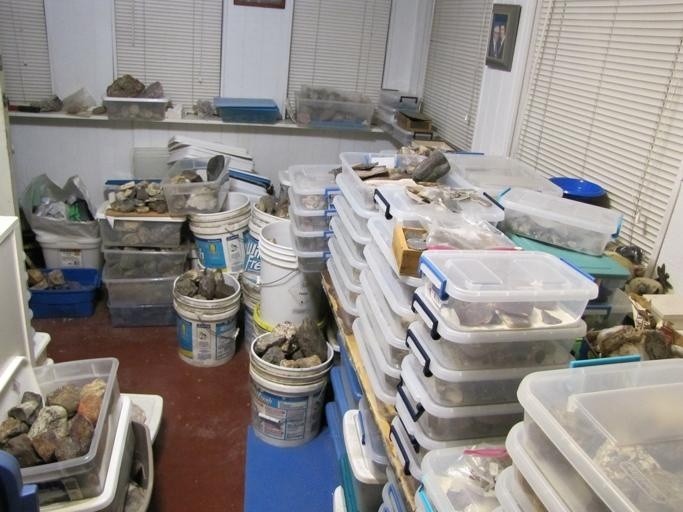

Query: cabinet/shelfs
(0, 217), (34, 373)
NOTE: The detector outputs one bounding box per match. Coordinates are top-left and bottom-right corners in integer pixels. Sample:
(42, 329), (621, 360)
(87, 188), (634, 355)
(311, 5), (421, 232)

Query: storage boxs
(27, 267), (100, 316)
(98, 157), (232, 328)
(376, 88), (437, 147)
(0, 331), (134, 511)
(36, 233), (102, 274)
(219, 106), (278, 124)
(101, 93), (170, 123)
(293, 84), (374, 132)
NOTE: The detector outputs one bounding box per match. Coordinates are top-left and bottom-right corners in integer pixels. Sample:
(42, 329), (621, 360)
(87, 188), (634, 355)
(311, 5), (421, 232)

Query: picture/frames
(484, 4), (520, 73)
(232, 1), (285, 10)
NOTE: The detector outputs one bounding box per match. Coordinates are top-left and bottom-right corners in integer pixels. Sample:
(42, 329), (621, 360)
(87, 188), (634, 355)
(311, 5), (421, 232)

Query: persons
(488, 25), (500, 59)
(498, 24), (506, 59)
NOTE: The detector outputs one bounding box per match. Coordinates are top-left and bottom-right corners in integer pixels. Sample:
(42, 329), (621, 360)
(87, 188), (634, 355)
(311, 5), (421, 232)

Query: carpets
(240, 425), (344, 510)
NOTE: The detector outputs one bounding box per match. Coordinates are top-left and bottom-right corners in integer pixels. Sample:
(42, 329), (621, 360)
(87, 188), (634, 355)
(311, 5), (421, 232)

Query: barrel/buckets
(195, 257), (241, 282)
(253, 301), (327, 338)
(171, 269), (242, 368)
(189, 191), (252, 272)
(248, 332), (336, 448)
(241, 283), (261, 353)
(242, 202), (284, 284)
(256, 220), (326, 326)
